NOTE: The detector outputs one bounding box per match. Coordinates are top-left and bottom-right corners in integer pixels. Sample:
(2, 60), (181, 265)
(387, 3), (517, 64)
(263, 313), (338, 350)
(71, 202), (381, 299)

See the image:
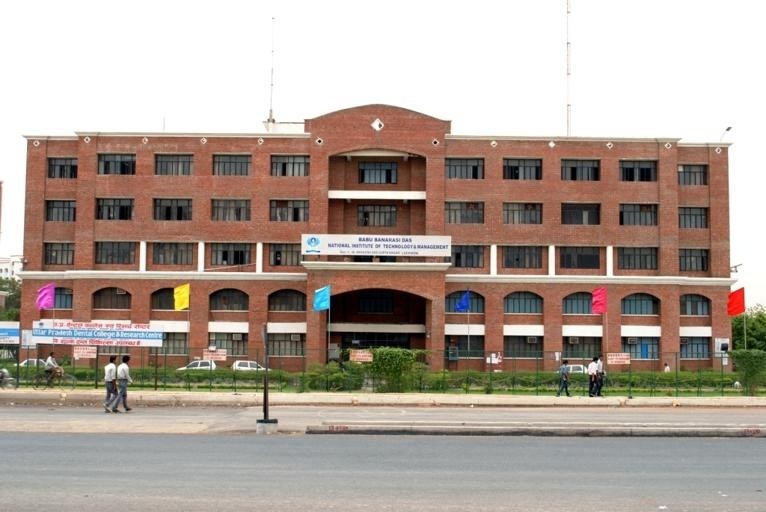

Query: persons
(111, 356), (133, 412)
(663, 362), (670, 374)
(44, 351), (61, 388)
(0, 366), (9, 388)
(590, 355), (606, 397)
(555, 360), (572, 397)
(587, 357), (599, 397)
(102, 354), (118, 414)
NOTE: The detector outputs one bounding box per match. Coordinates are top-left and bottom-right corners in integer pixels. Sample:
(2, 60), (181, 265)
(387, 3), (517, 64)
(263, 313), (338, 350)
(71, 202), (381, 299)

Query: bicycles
(0, 377), (17, 392)
(30, 366), (78, 393)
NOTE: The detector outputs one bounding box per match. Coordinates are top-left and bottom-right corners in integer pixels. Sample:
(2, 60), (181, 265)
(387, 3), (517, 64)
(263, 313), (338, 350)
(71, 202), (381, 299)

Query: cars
(173, 359), (217, 371)
(230, 360), (271, 371)
(555, 364), (608, 376)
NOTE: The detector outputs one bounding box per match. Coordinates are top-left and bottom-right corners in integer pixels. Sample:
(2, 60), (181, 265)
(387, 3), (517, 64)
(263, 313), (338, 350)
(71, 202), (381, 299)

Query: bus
(12, 357), (46, 367)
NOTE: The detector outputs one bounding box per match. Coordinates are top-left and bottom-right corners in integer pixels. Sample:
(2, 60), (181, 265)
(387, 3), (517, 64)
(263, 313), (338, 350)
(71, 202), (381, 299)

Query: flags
(727, 286), (747, 317)
(311, 284), (328, 312)
(453, 290), (470, 310)
(35, 283), (54, 311)
(173, 283), (188, 312)
(591, 287), (608, 314)
